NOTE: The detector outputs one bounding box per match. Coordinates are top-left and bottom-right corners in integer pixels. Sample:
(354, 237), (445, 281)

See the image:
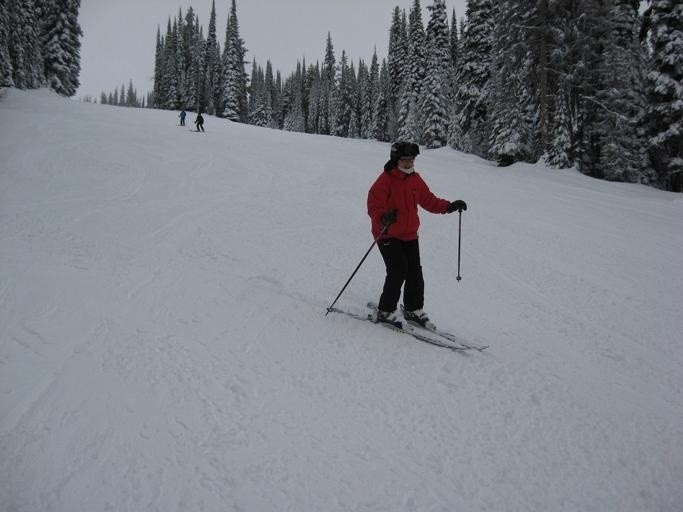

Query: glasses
(400, 159), (414, 163)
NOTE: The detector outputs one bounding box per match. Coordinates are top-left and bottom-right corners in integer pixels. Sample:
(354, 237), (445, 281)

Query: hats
(390, 141), (419, 159)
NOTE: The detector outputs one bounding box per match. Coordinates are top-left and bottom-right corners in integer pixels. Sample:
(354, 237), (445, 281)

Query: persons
(193, 112), (204, 133)
(366, 141), (465, 332)
(177, 108), (186, 126)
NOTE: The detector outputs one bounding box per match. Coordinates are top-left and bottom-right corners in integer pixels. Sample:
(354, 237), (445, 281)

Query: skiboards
(326, 302), (490, 351)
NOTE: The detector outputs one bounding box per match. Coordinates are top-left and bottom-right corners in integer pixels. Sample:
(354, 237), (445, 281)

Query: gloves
(447, 200), (467, 212)
(383, 209), (398, 223)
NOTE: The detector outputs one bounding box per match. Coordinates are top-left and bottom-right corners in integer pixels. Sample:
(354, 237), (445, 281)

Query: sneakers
(377, 312), (402, 328)
(404, 309), (429, 326)
